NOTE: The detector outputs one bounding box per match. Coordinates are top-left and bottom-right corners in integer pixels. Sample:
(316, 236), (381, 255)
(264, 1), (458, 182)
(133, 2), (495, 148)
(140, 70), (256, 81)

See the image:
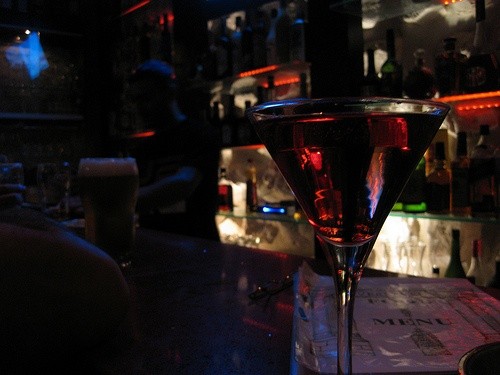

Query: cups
(231, 182), (247, 217)
(36, 161), (71, 219)
(77, 155), (142, 269)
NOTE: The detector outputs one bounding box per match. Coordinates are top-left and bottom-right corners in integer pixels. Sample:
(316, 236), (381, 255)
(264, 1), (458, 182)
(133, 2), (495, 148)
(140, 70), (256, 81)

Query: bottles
(403, 49), (437, 98)
(391, 156), (428, 214)
(469, 124), (500, 220)
(433, 38), (464, 95)
(244, 158), (260, 212)
(488, 258), (500, 291)
(441, 229), (467, 278)
(208, 0), (308, 78)
(218, 167), (233, 212)
(428, 141), (451, 215)
(449, 131), (476, 220)
(460, 0), (500, 98)
(464, 240), (490, 287)
(363, 47), (380, 94)
(380, 28), (402, 96)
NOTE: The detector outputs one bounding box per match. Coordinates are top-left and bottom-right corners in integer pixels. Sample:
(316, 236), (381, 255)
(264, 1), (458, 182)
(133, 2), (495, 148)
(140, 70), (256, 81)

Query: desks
(0, 227), (500, 375)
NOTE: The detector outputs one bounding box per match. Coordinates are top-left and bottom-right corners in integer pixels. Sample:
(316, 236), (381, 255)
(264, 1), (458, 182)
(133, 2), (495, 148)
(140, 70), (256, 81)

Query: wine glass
(245, 94), (452, 375)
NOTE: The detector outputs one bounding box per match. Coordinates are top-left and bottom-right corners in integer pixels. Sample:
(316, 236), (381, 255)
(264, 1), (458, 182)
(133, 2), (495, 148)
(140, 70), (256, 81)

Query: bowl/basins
(457, 341), (500, 375)
